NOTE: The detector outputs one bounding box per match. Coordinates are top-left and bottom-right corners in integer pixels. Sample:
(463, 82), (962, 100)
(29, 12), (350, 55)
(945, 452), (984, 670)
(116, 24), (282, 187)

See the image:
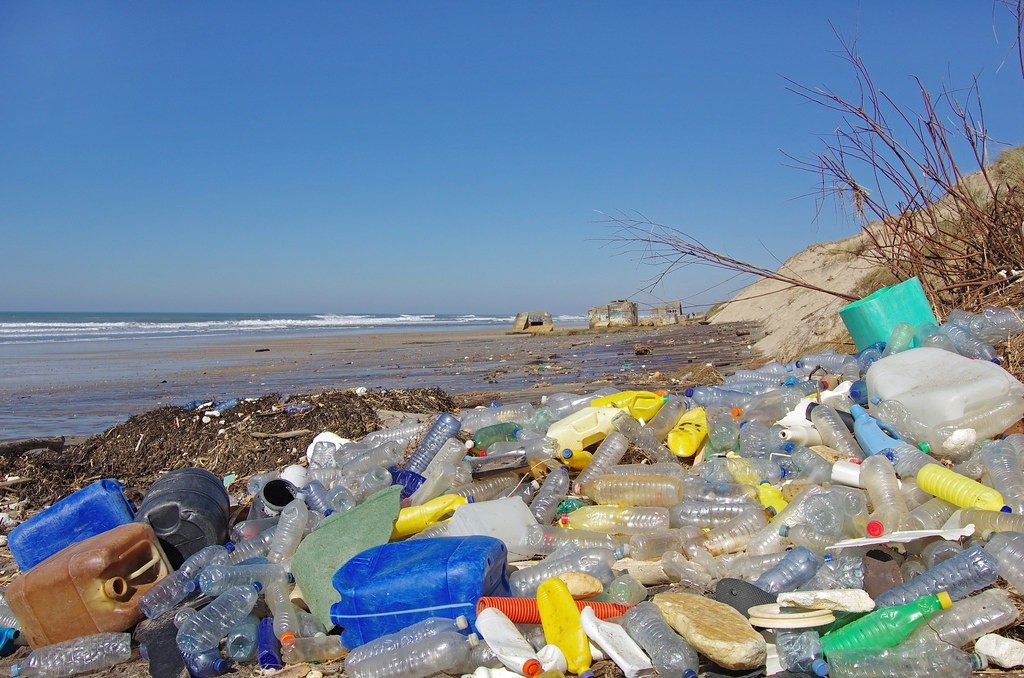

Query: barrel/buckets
(133, 468), (230, 568)
(545, 407), (633, 457)
(7, 479), (138, 574)
(4, 522), (174, 651)
(261, 479), (299, 517)
(867, 347), (1024, 452)
(332, 536), (513, 639)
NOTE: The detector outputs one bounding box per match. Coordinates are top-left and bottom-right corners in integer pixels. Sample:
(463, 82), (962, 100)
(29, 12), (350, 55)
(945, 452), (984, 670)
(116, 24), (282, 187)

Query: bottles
(0, 306), (1024, 678)
